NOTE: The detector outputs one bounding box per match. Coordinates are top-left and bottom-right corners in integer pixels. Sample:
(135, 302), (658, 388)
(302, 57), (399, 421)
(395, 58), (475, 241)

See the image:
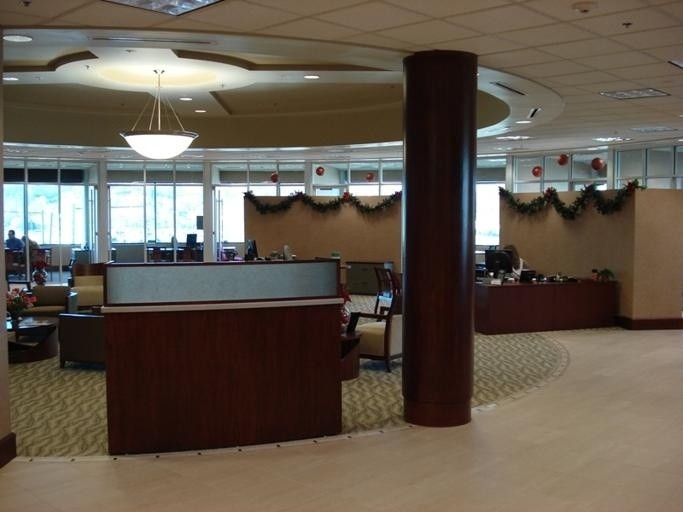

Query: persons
(20, 235), (45, 272)
(501, 244), (535, 280)
(4, 229), (23, 248)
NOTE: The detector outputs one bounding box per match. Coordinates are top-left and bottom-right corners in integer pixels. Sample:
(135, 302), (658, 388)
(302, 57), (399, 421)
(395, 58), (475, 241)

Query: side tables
(340, 332), (363, 381)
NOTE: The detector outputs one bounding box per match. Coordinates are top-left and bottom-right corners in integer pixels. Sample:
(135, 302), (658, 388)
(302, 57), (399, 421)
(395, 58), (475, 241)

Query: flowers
(33, 261), (49, 268)
(6, 288), (38, 319)
(339, 284), (353, 306)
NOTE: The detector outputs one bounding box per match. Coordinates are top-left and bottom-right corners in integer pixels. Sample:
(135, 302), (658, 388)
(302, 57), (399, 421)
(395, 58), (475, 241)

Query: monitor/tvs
(247, 239), (258, 257)
(485, 249), (512, 273)
(186, 234), (197, 244)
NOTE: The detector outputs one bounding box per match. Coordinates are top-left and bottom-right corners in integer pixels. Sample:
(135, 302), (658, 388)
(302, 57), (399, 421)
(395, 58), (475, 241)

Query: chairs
(20, 263), (105, 316)
(58, 314), (106, 372)
(346, 268), (403, 372)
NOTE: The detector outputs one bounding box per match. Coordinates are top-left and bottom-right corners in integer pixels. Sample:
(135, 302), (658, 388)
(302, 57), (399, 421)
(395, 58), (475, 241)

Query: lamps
(117, 70), (199, 160)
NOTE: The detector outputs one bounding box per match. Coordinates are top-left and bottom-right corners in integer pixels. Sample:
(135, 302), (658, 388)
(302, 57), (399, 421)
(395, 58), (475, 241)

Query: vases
(341, 306), (352, 336)
(6, 317), (24, 328)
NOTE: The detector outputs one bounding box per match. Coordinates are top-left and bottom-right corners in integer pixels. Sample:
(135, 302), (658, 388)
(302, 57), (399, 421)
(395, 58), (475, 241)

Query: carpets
(8, 295), (571, 464)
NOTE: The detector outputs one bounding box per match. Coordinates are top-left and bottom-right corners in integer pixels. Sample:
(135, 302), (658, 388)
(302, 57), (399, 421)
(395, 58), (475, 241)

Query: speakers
(197, 216), (203, 229)
